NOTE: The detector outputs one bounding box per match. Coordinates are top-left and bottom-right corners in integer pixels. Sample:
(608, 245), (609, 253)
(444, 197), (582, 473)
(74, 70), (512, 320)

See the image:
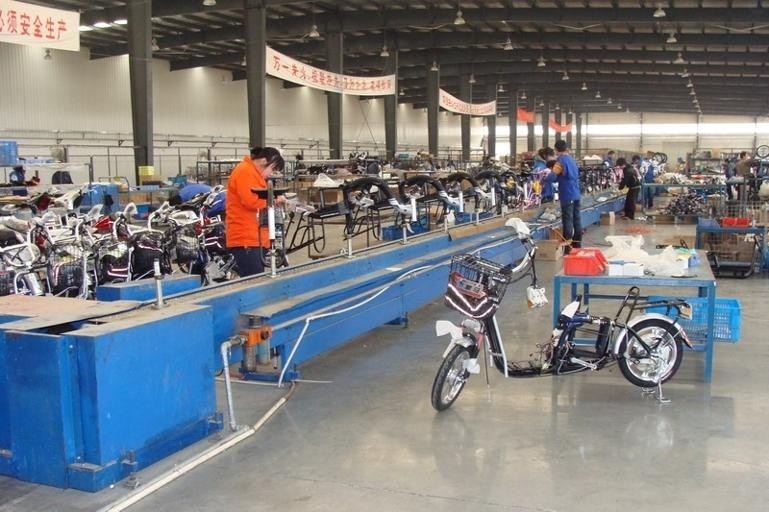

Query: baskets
(91, 223), (199, 275)
(448, 252), (512, 305)
(645, 295), (740, 344)
(50, 241), (84, 290)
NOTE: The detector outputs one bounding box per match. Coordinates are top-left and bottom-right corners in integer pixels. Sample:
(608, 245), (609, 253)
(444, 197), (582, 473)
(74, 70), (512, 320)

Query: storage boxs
(533, 239), (559, 262)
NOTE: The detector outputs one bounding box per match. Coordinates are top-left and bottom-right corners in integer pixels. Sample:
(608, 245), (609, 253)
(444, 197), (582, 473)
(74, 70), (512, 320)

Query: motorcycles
(0, 179), (287, 303)
(432, 235), (693, 414)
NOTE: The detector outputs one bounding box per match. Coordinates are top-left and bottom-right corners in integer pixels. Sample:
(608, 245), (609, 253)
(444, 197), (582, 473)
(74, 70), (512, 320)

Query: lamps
(79, 6), (128, 33)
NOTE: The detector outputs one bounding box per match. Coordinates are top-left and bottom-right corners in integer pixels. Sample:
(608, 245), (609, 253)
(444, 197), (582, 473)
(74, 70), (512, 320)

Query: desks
(552, 249), (718, 385)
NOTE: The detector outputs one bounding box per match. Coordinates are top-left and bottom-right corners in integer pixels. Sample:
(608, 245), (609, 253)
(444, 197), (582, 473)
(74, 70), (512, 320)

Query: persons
(723, 158), (734, 199)
(169, 182), (226, 216)
(542, 139), (582, 253)
(541, 158), (558, 204)
(225, 146), (285, 278)
(601, 149), (658, 220)
(532, 148), (556, 182)
(9, 156), (30, 197)
(734, 149), (759, 199)
